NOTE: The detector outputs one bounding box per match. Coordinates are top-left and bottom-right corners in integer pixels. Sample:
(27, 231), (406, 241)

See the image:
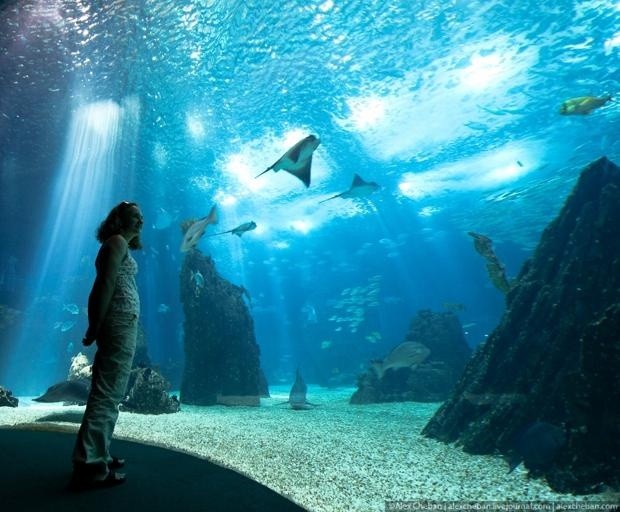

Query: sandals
(75, 457), (126, 487)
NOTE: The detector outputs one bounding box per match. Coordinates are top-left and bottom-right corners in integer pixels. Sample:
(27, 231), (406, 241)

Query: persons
(70, 201), (144, 486)
